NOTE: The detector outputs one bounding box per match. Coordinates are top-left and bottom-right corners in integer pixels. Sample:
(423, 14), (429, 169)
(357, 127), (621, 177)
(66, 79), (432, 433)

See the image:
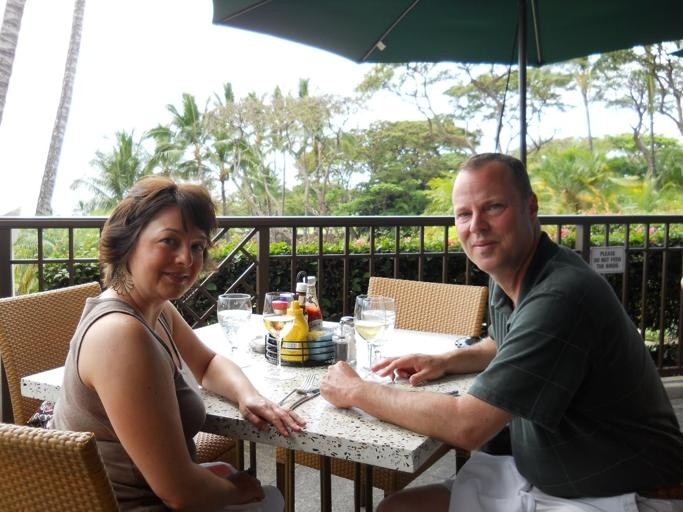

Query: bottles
(295, 275), (324, 333)
(331, 315), (358, 371)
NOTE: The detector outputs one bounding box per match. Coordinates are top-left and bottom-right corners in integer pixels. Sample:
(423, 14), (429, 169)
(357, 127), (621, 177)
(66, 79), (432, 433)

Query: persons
(319, 151), (682, 512)
(51, 174), (306, 512)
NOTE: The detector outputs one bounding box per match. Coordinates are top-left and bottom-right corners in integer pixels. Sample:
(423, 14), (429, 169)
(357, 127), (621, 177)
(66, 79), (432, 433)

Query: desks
(20, 311), (472, 512)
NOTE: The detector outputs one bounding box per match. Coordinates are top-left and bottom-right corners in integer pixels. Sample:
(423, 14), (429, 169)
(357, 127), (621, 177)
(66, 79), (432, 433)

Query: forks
(278, 374), (316, 406)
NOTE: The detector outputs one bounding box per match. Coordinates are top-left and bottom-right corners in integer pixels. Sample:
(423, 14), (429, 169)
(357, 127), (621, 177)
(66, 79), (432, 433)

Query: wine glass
(353, 293), (398, 385)
(261, 291), (298, 380)
(216, 293), (252, 369)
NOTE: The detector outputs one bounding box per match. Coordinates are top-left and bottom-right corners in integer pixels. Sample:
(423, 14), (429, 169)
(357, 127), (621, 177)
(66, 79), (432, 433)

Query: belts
(637, 484), (683, 501)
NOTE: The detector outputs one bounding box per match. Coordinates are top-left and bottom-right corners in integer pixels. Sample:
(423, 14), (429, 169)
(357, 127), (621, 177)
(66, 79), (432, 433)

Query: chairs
(273, 277), (487, 512)
(0, 419), (121, 512)
(2, 277), (247, 483)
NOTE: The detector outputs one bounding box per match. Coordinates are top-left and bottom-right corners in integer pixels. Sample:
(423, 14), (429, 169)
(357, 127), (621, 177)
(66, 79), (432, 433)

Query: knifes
(289, 388), (321, 410)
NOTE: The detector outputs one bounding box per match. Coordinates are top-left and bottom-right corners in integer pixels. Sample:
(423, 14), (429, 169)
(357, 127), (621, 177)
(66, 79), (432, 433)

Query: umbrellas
(210, 0), (682, 176)
